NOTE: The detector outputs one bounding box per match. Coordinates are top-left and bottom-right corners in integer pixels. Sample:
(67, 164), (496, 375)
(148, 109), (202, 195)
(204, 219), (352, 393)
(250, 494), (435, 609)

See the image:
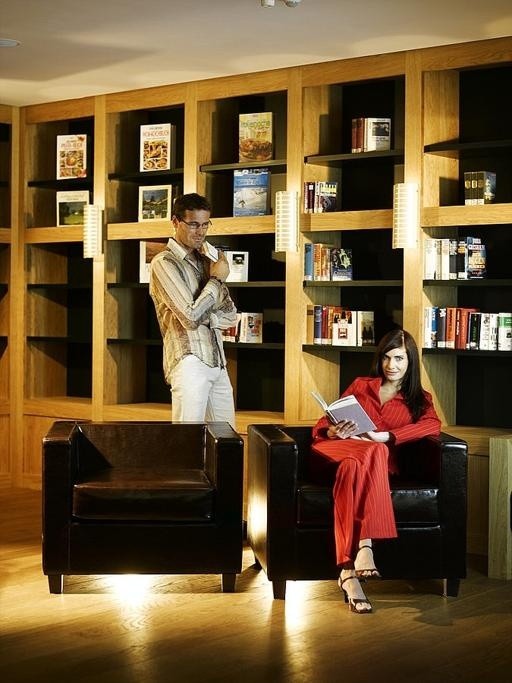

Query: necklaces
(388, 377), (405, 388)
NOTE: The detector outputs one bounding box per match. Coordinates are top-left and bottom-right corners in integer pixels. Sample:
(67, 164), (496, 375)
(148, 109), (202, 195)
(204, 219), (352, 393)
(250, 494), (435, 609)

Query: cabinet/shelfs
(0, 36), (512, 580)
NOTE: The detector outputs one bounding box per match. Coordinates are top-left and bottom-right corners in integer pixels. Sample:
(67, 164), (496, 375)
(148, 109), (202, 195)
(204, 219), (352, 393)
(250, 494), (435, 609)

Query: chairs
(249, 421), (468, 598)
(43, 421), (243, 593)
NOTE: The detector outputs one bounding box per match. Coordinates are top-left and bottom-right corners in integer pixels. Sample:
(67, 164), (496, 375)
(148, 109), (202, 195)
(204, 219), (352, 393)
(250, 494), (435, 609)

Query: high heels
(339, 576), (372, 613)
(354, 545), (380, 583)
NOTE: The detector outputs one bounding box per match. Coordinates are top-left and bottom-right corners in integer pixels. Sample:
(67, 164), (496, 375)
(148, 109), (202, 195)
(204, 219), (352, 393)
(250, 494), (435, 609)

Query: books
(56, 133), (88, 180)
(233, 169), (272, 216)
(217, 249), (249, 282)
(221, 312), (262, 343)
(424, 306), (512, 351)
(56, 189), (90, 227)
(304, 242), (352, 281)
(140, 241), (167, 283)
(306, 305), (375, 347)
(239, 114), (274, 162)
(465, 171), (496, 205)
(425, 237), (486, 281)
(351, 118), (391, 153)
(310, 390), (378, 438)
(304, 182), (338, 213)
(138, 185), (180, 222)
(140, 124), (175, 172)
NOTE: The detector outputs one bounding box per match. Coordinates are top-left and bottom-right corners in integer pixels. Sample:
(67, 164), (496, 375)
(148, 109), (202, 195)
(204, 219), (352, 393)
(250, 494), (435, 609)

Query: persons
(307, 329), (443, 614)
(148, 190), (242, 437)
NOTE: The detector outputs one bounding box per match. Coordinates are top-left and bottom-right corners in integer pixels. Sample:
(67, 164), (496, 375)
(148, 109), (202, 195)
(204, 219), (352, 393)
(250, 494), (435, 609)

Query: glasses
(182, 219), (212, 229)
